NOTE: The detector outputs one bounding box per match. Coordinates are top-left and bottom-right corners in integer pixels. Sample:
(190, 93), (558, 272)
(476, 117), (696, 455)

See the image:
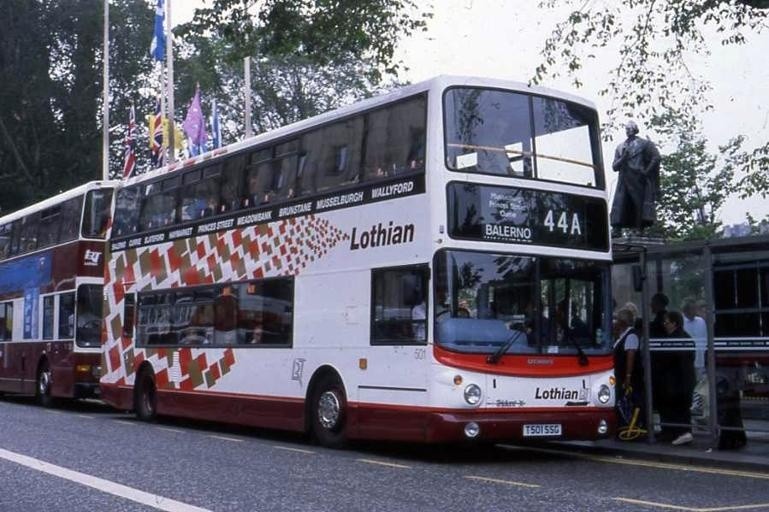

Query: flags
(148, 0), (165, 62)
(123, 80), (222, 180)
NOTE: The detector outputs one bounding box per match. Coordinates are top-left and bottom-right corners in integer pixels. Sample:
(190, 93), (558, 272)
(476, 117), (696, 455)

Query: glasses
(664, 321), (672, 326)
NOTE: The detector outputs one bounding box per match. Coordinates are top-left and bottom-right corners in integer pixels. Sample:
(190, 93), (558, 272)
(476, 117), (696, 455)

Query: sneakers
(672, 432), (693, 445)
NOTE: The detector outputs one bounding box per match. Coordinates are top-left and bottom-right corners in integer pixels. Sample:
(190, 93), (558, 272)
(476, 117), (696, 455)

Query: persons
(68, 299), (100, 336)
(411, 286), (452, 341)
(477, 117), (518, 175)
(612, 293), (709, 446)
(610, 120), (660, 239)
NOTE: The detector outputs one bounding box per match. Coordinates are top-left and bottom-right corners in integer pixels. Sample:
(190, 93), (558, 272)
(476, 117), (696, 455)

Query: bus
(0, 178), (118, 405)
(102, 69), (649, 453)
(134, 294), (291, 345)
